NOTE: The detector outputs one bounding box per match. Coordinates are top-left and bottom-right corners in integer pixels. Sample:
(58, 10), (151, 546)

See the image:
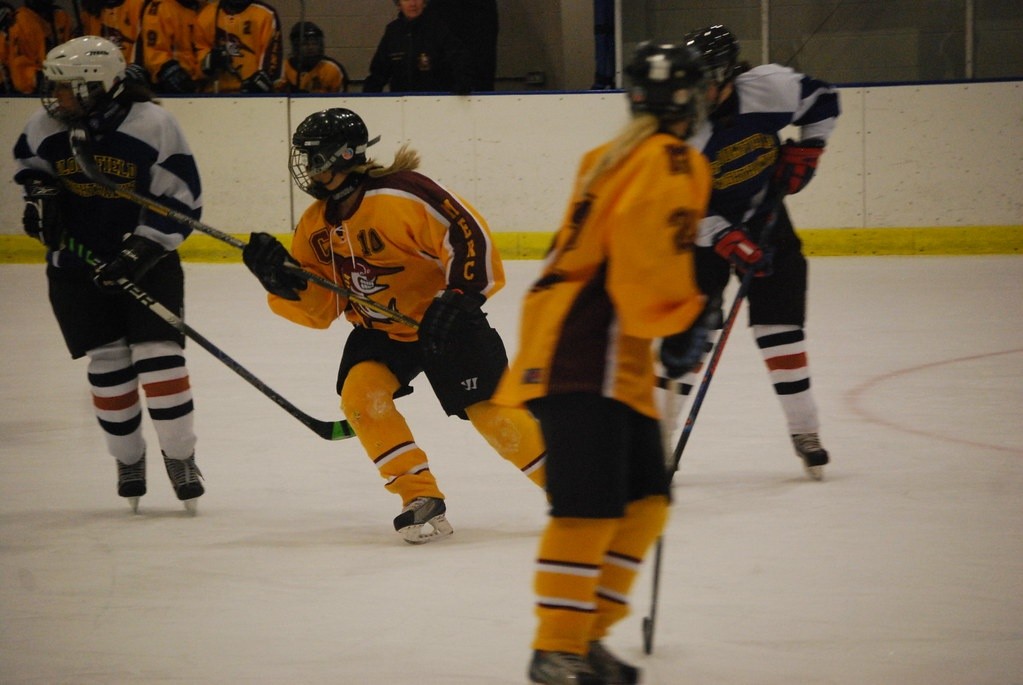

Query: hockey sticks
(70, 129), (421, 331)
(58, 234), (367, 441)
(654, 140), (792, 499)
(641, 535), (666, 653)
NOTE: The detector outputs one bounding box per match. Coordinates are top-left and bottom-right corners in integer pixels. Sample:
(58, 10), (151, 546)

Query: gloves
(713, 230), (774, 278)
(126, 63), (151, 85)
(156, 60), (196, 93)
(417, 286), (483, 357)
(775, 138), (825, 194)
(659, 293), (723, 378)
(14, 169), (66, 250)
(245, 70), (274, 93)
(243, 233), (307, 301)
(92, 232), (167, 295)
(201, 46), (233, 77)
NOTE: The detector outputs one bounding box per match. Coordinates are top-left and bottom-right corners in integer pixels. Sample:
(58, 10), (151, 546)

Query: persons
(503, 38), (713, 683)
(0, 0), (283, 98)
(658, 24), (840, 480)
(363, 0), (484, 91)
(242, 108), (547, 546)
(274, 21), (348, 94)
(13, 36), (205, 515)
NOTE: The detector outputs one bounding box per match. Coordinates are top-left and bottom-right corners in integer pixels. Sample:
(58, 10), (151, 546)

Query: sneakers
(161, 448), (206, 515)
(578, 640), (636, 685)
(792, 433), (828, 480)
(393, 496), (454, 544)
(528, 649), (603, 685)
(116, 444), (153, 513)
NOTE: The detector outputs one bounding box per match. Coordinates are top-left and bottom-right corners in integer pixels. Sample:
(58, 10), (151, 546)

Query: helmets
(624, 41), (701, 139)
(290, 21), (325, 68)
(286, 109), (368, 200)
(679, 26), (739, 86)
(41, 36), (127, 128)
